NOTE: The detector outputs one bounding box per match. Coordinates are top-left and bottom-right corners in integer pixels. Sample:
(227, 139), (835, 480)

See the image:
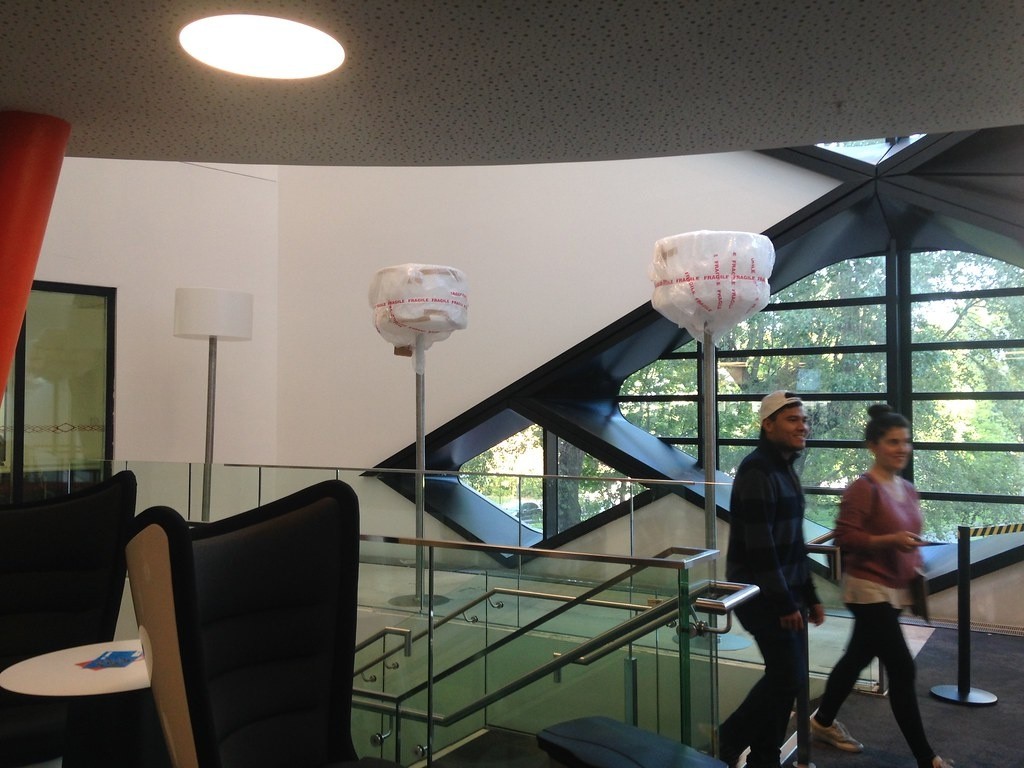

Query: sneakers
(932, 755), (954, 768)
(810, 706), (864, 752)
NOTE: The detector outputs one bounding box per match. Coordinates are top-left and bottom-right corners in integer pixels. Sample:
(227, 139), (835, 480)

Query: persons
(810, 404), (955, 767)
(715, 389), (825, 768)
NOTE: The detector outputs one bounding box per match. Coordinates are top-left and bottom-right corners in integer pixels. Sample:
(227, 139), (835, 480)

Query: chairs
(0, 468), (363, 768)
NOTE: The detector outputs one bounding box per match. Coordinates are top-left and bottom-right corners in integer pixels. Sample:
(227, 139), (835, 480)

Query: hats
(760, 389), (801, 421)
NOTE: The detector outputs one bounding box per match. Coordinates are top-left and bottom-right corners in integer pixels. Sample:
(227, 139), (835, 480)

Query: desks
(0, 639), (167, 768)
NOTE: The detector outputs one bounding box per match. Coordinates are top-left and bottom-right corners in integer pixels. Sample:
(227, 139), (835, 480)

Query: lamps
(170, 286), (255, 524)
